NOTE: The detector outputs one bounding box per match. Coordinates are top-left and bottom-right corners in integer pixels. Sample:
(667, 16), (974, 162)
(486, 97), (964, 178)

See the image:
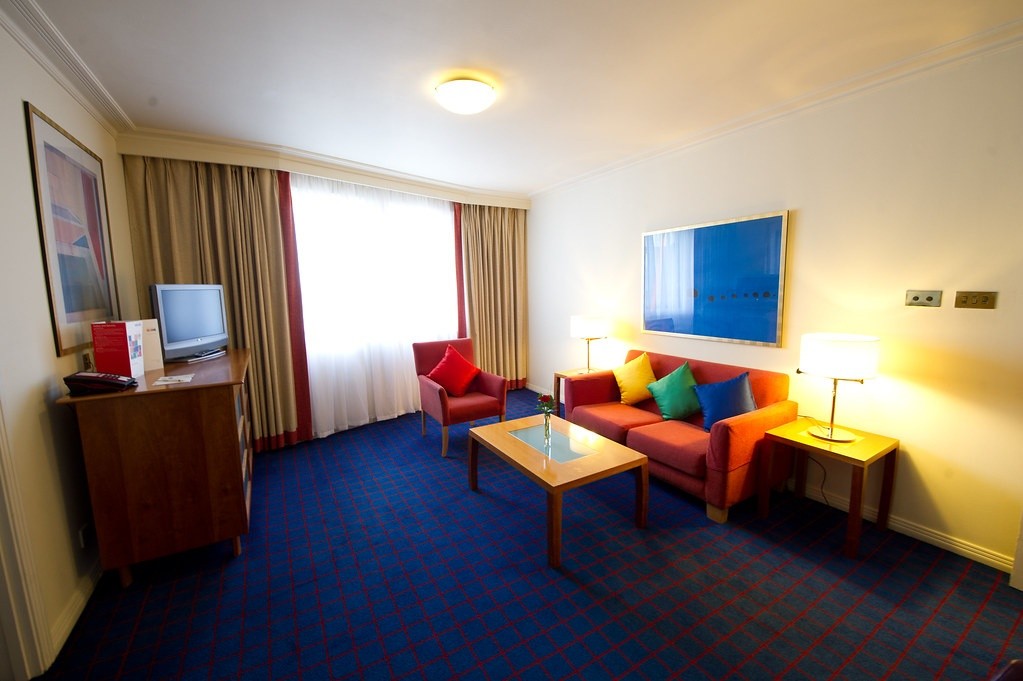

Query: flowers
(537, 393), (554, 417)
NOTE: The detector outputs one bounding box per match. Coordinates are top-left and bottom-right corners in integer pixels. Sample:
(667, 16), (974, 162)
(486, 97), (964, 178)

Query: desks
(759, 416), (900, 559)
(553, 369), (605, 418)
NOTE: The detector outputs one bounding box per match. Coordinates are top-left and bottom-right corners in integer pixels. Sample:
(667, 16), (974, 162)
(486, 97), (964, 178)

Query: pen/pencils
(155, 379), (184, 382)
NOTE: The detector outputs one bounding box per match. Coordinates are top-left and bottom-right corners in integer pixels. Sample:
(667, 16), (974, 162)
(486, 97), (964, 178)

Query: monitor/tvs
(148, 284), (228, 363)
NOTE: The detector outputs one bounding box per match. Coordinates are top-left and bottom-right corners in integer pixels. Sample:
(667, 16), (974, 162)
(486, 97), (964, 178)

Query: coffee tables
(469, 412), (648, 568)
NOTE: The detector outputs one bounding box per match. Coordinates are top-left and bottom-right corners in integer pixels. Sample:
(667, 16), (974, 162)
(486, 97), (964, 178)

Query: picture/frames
(639, 210), (789, 348)
(25, 100), (123, 360)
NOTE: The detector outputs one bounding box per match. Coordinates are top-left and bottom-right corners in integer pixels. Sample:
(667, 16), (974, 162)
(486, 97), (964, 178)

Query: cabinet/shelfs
(57, 346), (253, 586)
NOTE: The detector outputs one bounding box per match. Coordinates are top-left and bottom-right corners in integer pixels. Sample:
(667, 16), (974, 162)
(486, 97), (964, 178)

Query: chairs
(412, 337), (508, 457)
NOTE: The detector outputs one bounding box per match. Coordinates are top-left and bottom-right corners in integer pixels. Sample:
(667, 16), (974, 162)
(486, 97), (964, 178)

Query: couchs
(566, 349), (798, 521)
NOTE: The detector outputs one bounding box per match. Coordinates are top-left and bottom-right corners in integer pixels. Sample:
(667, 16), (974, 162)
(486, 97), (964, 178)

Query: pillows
(647, 360), (702, 420)
(611, 352), (659, 405)
(693, 371), (757, 432)
(426, 344), (479, 397)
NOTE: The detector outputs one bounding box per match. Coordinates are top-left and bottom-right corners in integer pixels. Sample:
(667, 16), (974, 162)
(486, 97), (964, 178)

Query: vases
(543, 412), (551, 438)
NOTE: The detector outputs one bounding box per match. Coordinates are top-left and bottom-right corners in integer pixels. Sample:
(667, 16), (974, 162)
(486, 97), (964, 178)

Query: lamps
(434, 78), (497, 114)
(799, 331), (878, 442)
(572, 314), (611, 373)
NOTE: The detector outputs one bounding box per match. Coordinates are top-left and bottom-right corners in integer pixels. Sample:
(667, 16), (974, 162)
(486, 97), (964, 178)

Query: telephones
(63, 370), (138, 398)
